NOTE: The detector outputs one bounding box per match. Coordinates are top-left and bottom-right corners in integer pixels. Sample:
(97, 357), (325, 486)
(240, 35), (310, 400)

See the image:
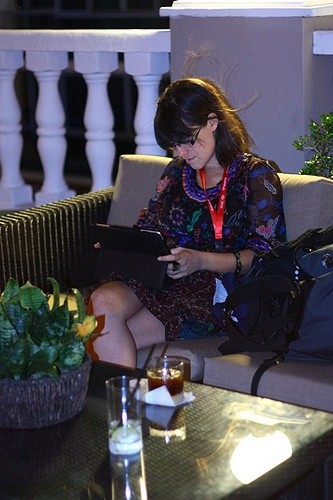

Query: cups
(103, 375), (146, 455)
(109, 456), (149, 500)
(146, 402), (186, 437)
(148, 356), (185, 401)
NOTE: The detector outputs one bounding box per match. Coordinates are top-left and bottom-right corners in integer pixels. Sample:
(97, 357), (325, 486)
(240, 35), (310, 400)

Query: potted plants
(0, 277), (109, 430)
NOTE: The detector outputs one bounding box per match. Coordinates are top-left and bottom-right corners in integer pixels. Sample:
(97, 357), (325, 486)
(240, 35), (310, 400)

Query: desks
(1, 360), (333, 500)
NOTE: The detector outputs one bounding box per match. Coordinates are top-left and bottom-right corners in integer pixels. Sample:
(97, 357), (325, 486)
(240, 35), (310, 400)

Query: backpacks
(217, 225), (333, 356)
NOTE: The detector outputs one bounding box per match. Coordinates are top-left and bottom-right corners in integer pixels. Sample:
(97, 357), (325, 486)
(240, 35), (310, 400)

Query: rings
(171, 261), (180, 271)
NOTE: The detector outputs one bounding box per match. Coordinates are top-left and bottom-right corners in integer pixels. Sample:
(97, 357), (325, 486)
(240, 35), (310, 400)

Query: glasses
(167, 122), (204, 151)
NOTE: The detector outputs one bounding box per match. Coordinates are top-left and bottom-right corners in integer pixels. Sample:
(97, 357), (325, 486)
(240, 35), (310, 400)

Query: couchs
(0, 186), (333, 412)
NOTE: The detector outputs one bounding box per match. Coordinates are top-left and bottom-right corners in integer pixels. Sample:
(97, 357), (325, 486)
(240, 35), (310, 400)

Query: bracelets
(232, 247), (251, 275)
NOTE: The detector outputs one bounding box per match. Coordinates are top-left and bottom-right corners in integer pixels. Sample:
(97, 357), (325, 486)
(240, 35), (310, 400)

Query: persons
(85, 76), (285, 368)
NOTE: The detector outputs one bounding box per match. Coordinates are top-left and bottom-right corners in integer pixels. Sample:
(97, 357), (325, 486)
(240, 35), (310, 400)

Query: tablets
(87, 223), (178, 289)
